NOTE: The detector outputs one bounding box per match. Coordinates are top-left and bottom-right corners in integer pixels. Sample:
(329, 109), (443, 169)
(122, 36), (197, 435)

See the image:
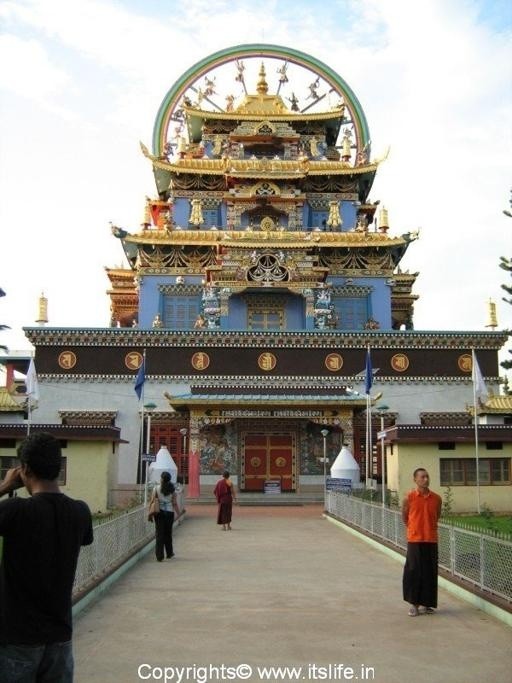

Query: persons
(1, 433), (93, 682)
(213, 470), (236, 529)
(400, 467), (443, 616)
(147, 471), (181, 561)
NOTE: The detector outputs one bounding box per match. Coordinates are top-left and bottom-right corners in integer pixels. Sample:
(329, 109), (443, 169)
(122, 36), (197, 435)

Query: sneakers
(407, 604), (435, 617)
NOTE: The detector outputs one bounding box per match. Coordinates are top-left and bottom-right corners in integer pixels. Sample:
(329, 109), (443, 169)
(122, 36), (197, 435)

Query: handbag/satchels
(149, 494), (161, 515)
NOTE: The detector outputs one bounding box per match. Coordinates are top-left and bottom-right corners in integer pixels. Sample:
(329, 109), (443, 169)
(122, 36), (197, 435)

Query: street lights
(143, 402), (156, 503)
(345, 387), (383, 478)
(179, 428), (188, 485)
(321, 429), (329, 503)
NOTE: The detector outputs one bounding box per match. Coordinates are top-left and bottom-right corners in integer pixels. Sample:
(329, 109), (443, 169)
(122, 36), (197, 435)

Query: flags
(24, 355), (41, 404)
(364, 348), (372, 394)
(134, 354), (145, 399)
(472, 349), (488, 407)
